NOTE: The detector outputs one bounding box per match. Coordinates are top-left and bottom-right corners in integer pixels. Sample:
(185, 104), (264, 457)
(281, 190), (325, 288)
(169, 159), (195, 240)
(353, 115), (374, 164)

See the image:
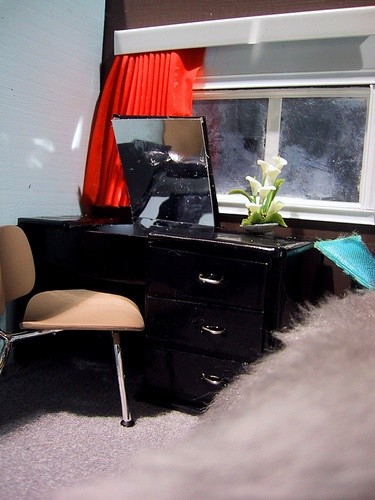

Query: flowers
(226, 154), (288, 228)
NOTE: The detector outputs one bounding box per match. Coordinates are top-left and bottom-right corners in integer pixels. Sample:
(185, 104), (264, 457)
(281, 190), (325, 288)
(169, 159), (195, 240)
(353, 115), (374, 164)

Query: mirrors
(110, 113), (221, 231)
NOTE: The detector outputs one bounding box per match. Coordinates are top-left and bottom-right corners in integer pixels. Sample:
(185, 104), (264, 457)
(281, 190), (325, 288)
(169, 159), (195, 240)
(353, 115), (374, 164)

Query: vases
(240, 223), (279, 234)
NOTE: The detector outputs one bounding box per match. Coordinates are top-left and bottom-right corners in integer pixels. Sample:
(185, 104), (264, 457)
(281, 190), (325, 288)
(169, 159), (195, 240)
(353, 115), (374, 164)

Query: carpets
(0, 360), (206, 500)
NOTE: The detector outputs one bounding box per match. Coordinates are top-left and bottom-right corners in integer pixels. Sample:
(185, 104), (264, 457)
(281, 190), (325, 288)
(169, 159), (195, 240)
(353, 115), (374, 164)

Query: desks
(18, 213), (326, 412)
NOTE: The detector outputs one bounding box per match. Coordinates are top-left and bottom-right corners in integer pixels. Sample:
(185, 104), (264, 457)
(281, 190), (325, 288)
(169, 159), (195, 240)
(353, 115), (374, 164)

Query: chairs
(0, 224), (144, 428)
